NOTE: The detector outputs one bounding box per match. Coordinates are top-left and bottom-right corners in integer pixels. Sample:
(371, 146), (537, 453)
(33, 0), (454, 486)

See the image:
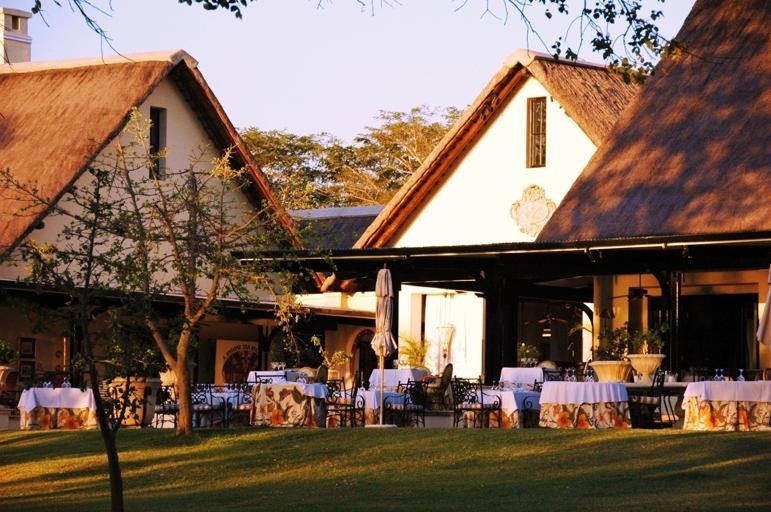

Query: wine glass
(360, 381), (377, 392)
(270, 361), (286, 371)
(563, 368), (577, 381)
(712, 367), (726, 382)
(296, 370), (307, 384)
(193, 382), (246, 393)
(43, 380), (53, 389)
(393, 359), (407, 369)
(519, 357), (540, 368)
(62, 377), (71, 388)
(583, 369), (595, 383)
(736, 368), (746, 381)
(490, 379), (534, 391)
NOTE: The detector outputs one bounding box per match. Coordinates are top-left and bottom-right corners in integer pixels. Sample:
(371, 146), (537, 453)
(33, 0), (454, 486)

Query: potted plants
(514, 342), (538, 364)
(587, 326), (631, 381)
(624, 321), (673, 380)
(309, 336), (352, 383)
(109, 348), (162, 428)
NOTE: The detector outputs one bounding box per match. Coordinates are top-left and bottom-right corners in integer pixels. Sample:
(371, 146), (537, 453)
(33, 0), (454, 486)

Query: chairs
(16, 364), (771, 431)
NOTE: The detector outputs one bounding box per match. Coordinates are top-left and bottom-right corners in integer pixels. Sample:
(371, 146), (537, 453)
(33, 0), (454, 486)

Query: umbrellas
(370, 261), (399, 425)
